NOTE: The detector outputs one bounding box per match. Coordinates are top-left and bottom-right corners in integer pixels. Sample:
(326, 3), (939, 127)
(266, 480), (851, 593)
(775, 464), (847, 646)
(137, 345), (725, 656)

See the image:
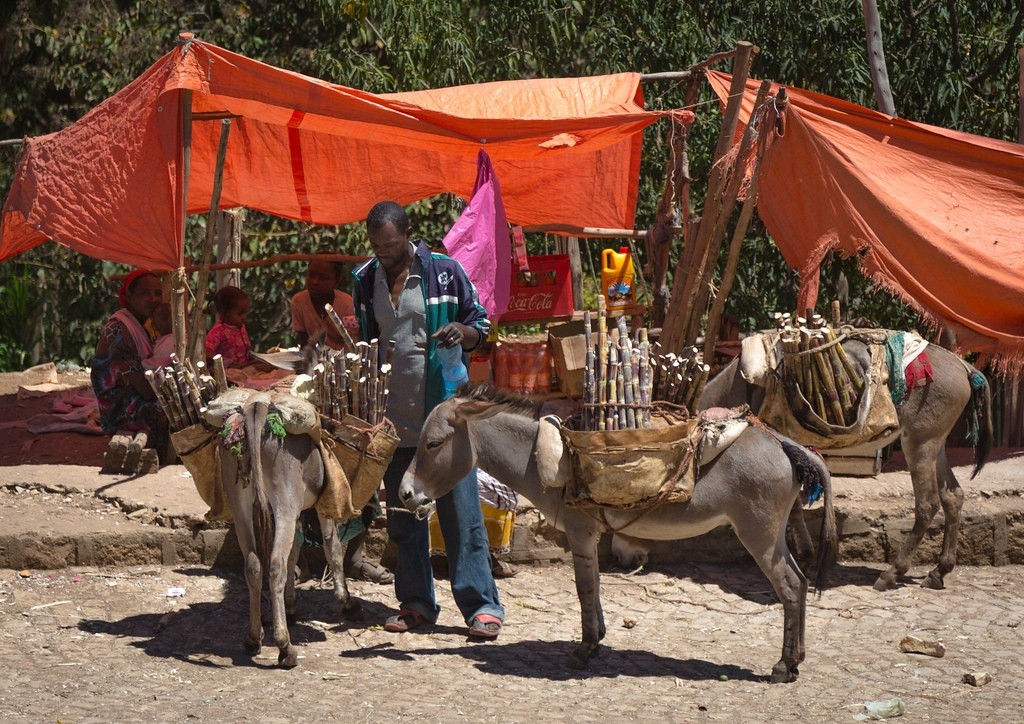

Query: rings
(449, 337), (456, 343)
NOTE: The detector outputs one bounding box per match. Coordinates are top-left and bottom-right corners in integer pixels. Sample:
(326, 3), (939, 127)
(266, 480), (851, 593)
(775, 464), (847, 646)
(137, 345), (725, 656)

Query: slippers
(343, 556), (395, 584)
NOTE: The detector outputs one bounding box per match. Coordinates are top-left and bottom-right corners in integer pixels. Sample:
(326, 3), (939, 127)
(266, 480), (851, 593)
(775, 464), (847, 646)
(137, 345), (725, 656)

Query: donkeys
(398, 379), (840, 684)
(215, 373), (364, 670)
(699, 327), (994, 590)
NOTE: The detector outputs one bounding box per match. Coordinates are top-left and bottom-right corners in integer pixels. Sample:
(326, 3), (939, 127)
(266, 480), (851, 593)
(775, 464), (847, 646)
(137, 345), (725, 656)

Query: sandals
(469, 616), (502, 636)
(385, 608), (422, 631)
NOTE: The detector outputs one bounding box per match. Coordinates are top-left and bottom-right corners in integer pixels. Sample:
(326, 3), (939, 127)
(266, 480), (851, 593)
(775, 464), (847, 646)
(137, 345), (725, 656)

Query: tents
(0, 30), (1024, 373)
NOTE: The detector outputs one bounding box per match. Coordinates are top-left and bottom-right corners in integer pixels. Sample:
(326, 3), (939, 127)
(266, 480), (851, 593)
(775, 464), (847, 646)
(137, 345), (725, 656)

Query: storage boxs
(465, 253), (622, 401)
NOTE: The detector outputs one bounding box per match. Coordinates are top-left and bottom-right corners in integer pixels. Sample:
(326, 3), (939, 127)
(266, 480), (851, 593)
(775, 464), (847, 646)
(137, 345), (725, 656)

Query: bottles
(436, 340), (470, 397)
(488, 268), (559, 396)
(601, 246), (637, 311)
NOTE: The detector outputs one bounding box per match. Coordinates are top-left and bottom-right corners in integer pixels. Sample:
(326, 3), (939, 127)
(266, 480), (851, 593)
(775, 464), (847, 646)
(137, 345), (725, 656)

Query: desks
(494, 304), (648, 342)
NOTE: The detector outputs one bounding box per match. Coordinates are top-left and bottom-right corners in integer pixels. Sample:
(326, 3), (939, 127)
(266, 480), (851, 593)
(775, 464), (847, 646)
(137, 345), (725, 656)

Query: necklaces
(314, 298), (328, 319)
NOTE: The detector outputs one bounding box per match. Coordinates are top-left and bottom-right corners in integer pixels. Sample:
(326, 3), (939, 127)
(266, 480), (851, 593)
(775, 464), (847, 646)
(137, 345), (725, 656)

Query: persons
(288, 250), (356, 351)
(91, 264), (252, 465)
(351, 201), (503, 638)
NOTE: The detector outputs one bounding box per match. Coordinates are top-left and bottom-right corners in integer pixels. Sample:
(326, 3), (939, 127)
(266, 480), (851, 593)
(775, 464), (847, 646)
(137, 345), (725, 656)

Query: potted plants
(706, 283), (764, 342)
